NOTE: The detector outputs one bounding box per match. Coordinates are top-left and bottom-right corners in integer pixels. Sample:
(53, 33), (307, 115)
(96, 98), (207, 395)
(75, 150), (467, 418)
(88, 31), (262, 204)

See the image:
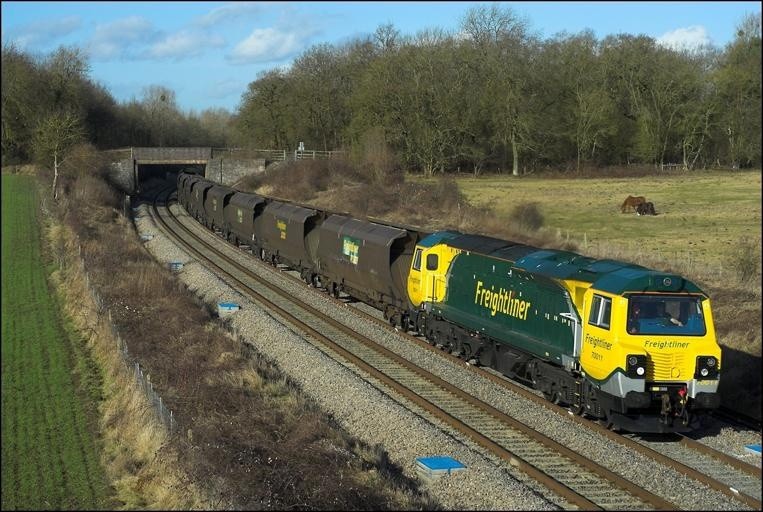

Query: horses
(621, 195), (645, 214)
(636, 202), (655, 215)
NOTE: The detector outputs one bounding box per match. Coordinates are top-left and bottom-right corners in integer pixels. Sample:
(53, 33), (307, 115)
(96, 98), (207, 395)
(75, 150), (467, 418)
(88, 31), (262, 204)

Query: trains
(175, 164), (724, 439)
